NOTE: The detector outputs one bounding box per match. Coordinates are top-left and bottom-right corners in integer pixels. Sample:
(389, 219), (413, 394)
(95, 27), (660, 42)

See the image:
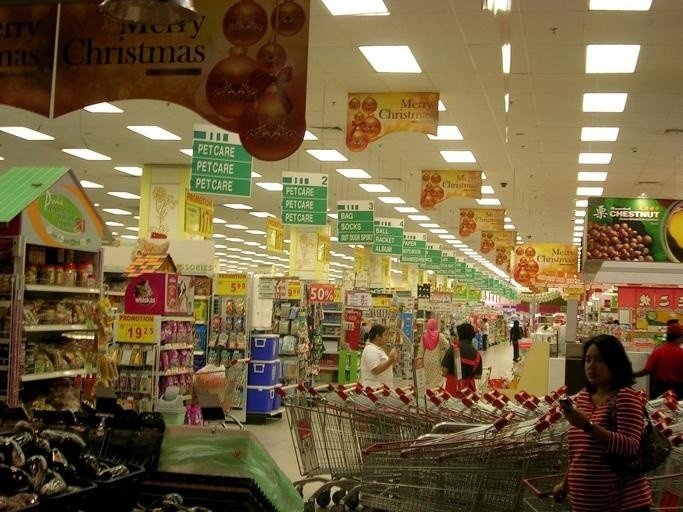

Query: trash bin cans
(158, 386), (187, 425)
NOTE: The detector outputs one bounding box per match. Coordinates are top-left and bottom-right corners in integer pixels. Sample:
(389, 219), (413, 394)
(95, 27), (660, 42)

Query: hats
(667, 324), (683, 336)
(455, 323), (476, 339)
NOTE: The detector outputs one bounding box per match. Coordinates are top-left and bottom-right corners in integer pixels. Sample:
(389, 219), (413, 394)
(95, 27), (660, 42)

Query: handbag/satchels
(415, 358), (424, 369)
(605, 386), (673, 479)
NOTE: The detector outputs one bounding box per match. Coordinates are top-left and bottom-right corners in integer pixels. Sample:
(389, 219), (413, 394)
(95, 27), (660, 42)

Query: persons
(360, 322), (370, 345)
(440, 324), (482, 399)
(360, 326), (401, 389)
(553, 335), (654, 512)
(481, 318), (490, 351)
(417, 318), (450, 400)
(666, 319), (680, 326)
(631, 319), (683, 402)
(510, 320), (523, 361)
(614, 320), (619, 326)
(605, 316), (613, 324)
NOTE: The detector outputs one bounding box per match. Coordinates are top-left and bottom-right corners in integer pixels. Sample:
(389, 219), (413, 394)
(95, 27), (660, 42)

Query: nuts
(586, 221), (654, 261)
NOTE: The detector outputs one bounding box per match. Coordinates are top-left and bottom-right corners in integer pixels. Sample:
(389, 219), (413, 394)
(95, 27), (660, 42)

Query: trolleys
(442, 367), (491, 397)
(193, 358), (251, 430)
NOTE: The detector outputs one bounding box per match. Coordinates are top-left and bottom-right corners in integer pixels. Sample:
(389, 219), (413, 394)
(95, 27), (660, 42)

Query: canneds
(25, 247), (98, 288)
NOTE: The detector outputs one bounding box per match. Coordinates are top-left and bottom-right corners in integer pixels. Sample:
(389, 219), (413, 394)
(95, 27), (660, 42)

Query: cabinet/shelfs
(248, 275), (324, 420)
(0, 236), (106, 422)
(104, 270), (254, 416)
(308, 282), (436, 388)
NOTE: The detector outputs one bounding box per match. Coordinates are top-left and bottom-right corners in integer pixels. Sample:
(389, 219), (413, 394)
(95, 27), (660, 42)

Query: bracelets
(584, 421), (594, 435)
